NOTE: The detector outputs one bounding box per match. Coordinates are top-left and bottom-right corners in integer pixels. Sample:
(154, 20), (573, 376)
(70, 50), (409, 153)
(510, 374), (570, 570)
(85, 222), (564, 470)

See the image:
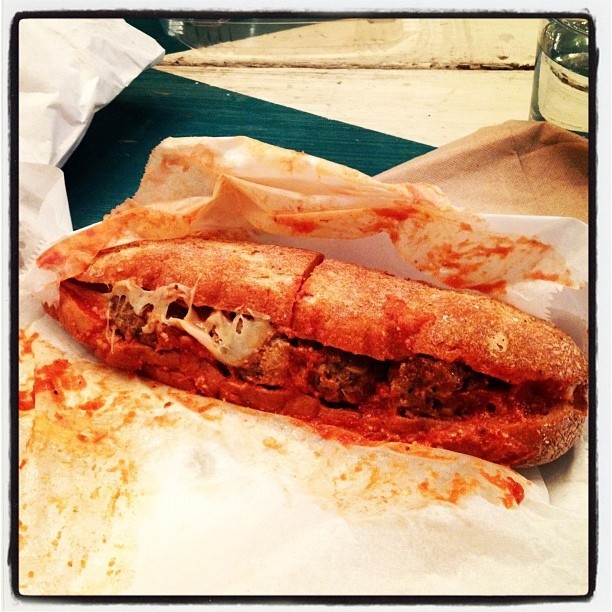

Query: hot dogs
(41, 237), (587, 467)
